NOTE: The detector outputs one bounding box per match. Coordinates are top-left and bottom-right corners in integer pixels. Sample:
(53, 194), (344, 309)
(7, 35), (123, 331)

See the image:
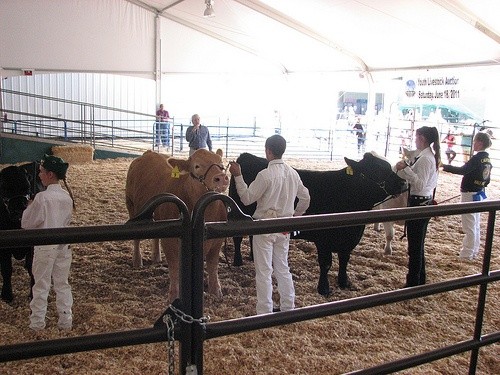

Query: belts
(410, 196), (431, 199)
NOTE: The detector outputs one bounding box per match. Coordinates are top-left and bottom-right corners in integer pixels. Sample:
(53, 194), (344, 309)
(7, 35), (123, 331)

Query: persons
(229, 135), (310, 315)
(351, 119), (366, 155)
(156, 104), (170, 148)
(21, 155), (74, 331)
(396, 126), (442, 288)
(441, 129), (456, 165)
(186, 114), (212, 157)
(440, 132), (492, 260)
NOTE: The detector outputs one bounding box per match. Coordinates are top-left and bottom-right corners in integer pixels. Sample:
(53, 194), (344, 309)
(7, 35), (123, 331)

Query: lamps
(204, 0), (216, 18)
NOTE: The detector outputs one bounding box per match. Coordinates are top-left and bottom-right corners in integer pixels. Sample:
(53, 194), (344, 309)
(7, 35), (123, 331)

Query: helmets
(41, 153), (69, 179)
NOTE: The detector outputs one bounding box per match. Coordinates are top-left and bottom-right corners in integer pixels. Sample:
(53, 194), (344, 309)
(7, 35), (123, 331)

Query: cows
(126, 149), (230, 304)
(374, 158), (411, 253)
(227, 152), (407, 298)
(0, 162), (47, 302)
